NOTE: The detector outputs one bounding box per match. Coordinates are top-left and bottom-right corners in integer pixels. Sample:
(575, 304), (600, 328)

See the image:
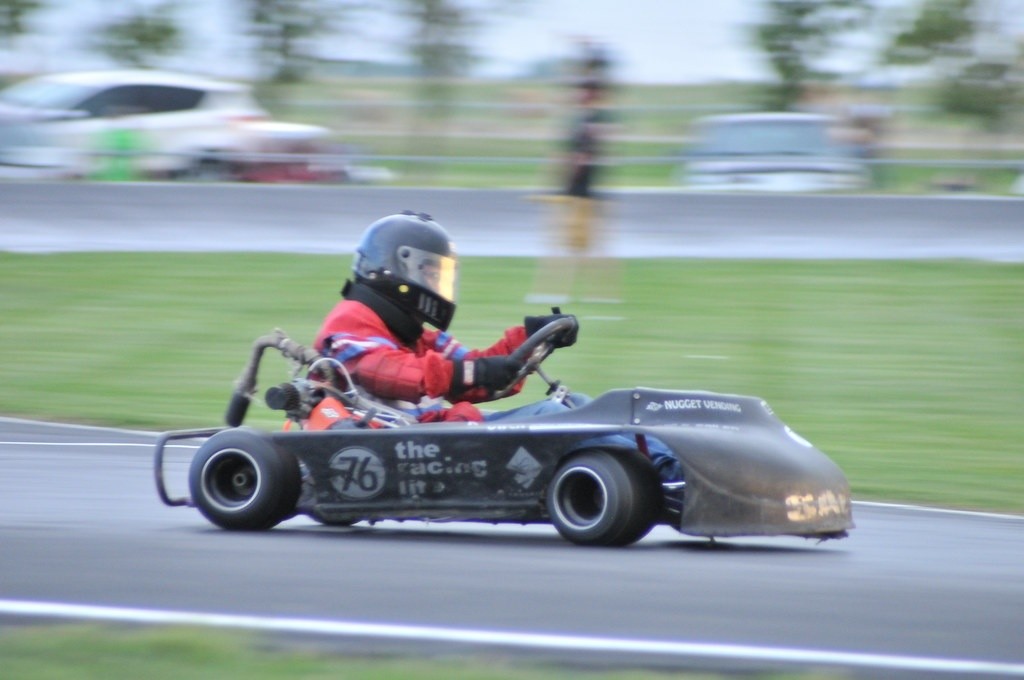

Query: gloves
(524, 307), (578, 348)
(451, 354), (522, 396)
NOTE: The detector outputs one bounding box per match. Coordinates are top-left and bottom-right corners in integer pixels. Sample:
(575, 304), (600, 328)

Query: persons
(303, 214), (593, 428)
(559, 51), (611, 193)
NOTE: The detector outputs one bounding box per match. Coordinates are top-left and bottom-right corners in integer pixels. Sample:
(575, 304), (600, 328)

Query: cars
(1, 70), (270, 177)
(681, 109), (877, 191)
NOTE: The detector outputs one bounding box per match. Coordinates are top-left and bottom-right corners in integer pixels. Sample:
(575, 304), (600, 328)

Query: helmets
(354, 210), (456, 331)
